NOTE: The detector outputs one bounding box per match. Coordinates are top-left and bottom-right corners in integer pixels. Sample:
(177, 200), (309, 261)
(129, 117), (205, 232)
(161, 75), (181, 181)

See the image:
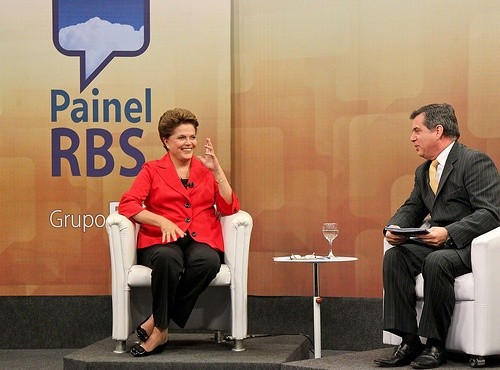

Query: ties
(429, 159), (439, 196)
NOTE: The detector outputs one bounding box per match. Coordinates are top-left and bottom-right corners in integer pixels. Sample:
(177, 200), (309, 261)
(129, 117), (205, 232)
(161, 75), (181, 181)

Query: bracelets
(216, 176), (226, 184)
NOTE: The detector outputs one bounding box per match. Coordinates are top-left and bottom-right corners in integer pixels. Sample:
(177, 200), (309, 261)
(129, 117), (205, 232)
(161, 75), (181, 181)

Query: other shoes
(137, 314), (152, 342)
(130, 338), (169, 357)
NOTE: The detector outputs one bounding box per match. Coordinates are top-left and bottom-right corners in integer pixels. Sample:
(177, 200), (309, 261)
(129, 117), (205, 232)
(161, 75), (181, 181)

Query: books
(386, 227), (429, 237)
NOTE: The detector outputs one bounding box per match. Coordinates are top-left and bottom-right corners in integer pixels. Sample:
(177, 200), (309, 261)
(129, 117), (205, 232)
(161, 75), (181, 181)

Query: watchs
(444, 233), (454, 246)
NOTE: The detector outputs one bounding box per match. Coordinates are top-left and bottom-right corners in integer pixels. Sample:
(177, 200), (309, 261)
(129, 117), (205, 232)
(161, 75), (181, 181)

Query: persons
(119, 108), (240, 356)
(374, 103), (500, 367)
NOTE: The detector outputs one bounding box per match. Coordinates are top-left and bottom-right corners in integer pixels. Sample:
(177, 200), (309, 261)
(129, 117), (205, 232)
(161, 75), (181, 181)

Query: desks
(273, 256), (358, 359)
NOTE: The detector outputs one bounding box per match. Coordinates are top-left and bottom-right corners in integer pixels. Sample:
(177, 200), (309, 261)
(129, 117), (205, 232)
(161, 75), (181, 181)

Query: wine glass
(322, 222), (339, 260)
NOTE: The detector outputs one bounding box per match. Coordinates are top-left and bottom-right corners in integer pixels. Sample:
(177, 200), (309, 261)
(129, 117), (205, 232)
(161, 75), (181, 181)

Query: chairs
(105, 210), (253, 352)
(381, 226), (500, 367)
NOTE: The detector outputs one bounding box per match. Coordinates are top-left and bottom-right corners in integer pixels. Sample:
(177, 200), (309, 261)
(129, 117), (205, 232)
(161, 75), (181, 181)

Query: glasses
(290, 251), (316, 260)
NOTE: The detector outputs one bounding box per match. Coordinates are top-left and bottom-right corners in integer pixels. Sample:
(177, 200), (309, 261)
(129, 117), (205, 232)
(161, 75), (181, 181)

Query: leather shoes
(410, 348), (448, 368)
(375, 343), (425, 366)
